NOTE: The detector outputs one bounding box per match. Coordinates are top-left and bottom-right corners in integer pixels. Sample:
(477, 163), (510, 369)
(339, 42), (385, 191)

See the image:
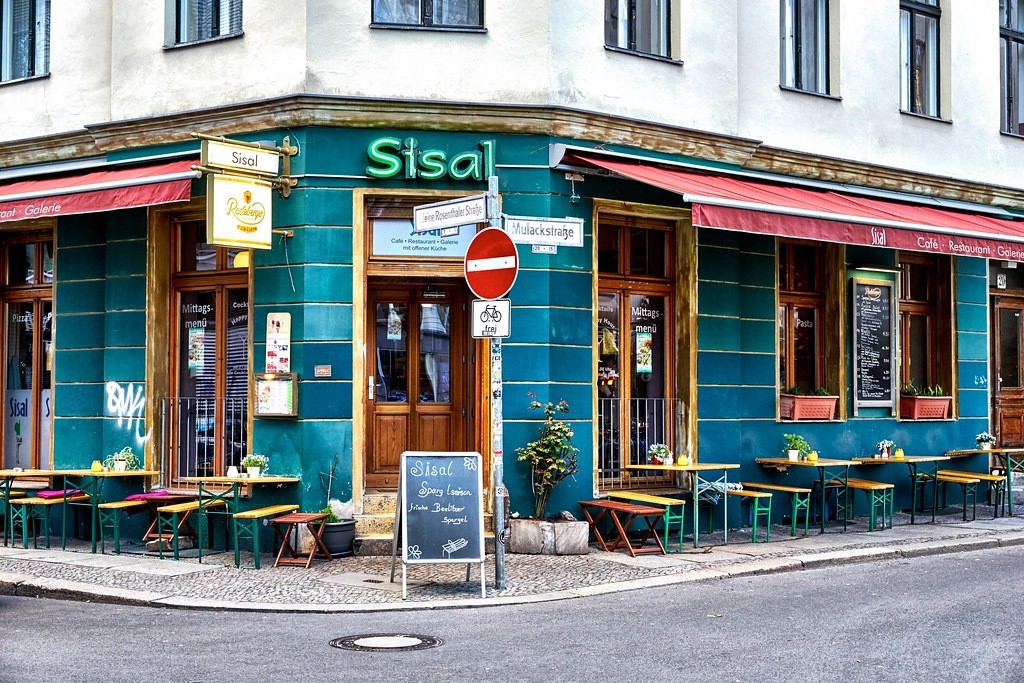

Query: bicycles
(480, 303), (503, 323)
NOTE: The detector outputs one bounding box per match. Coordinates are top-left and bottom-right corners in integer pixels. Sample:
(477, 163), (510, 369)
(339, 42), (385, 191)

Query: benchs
(728, 482), (812, 543)
(233, 505), (300, 569)
(98, 501), (148, 555)
(157, 500), (229, 560)
(0, 492), (100, 548)
(909, 466), (1023, 521)
(578, 491), (686, 558)
(814, 478), (895, 532)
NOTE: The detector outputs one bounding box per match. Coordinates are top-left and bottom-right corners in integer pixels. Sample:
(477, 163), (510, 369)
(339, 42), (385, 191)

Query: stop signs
(463, 226), (519, 302)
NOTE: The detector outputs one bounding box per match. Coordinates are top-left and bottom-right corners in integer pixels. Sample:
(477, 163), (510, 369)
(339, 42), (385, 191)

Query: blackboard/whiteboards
(392, 450), (486, 564)
(850, 277), (896, 408)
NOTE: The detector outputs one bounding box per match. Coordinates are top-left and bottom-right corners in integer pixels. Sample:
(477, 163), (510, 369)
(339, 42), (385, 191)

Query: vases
(247, 466), (260, 478)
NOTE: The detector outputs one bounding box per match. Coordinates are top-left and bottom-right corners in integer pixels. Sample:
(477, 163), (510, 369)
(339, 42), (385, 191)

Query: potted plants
(780, 433), (811, 463)
(311, 504), (358, 559)
(102, 446), (140, 471)
(509, 391), (589, 555)
(780, 384), (839, 420)
(900, 377), (953, 420)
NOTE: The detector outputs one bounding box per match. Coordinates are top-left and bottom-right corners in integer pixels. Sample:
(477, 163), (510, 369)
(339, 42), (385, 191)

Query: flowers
(975, 431), (996, 450)
(876, 439), (896, 458)
(646, 443), (673, 465)
(240, 454), (270, 477)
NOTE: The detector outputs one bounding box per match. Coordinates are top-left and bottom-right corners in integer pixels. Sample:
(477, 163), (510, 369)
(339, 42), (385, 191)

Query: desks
(269, 513), (333, 569)
(184, 477), (298, 569)
(0, 470), (160, 554)
(851, 456), (951, 525)
(755, 458), (862, 534)
(946, 449), (1024, 516)
(626, 463), (741, 549)
(142, 495), (199, 542)
